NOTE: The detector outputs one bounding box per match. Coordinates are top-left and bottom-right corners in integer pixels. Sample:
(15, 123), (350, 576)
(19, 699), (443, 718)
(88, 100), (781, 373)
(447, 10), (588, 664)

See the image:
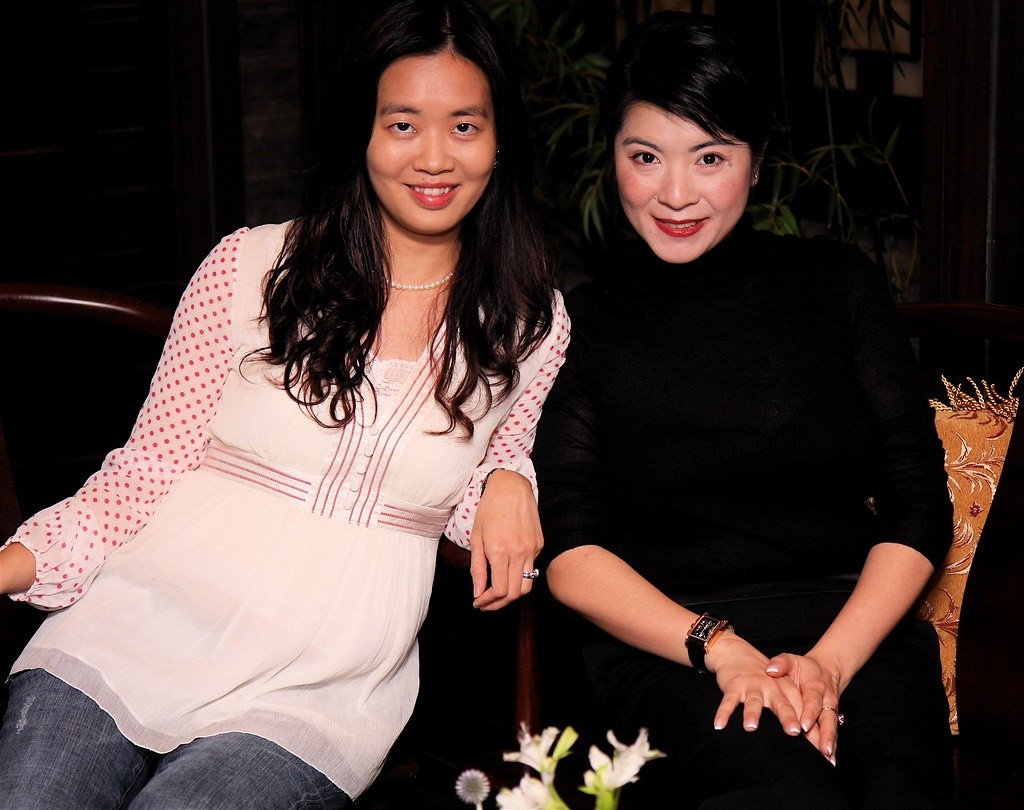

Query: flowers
(456, 723), (669, 810)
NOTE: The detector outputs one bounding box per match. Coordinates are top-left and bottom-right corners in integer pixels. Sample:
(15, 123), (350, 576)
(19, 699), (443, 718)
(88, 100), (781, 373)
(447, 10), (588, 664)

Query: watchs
(684, 612), (728, 677)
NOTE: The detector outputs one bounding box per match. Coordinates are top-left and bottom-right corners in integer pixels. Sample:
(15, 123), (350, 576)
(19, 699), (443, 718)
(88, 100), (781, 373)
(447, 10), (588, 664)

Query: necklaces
(375, 272), (453, 290)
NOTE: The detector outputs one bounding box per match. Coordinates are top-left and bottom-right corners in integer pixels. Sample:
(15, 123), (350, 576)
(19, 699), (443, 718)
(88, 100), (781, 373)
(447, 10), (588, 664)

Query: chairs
(0, 280), (538, 809)
(891, 299), (1024, 810)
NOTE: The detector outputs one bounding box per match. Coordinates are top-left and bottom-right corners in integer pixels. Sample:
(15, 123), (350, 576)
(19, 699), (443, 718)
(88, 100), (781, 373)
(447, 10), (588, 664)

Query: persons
(461, 7), (955, 810)
(0, 0), (571, 810)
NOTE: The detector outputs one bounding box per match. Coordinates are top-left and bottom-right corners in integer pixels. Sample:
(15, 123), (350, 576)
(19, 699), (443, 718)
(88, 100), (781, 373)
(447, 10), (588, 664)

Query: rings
(523, 569), (540, 577)
(820, 705), (844, 726)
